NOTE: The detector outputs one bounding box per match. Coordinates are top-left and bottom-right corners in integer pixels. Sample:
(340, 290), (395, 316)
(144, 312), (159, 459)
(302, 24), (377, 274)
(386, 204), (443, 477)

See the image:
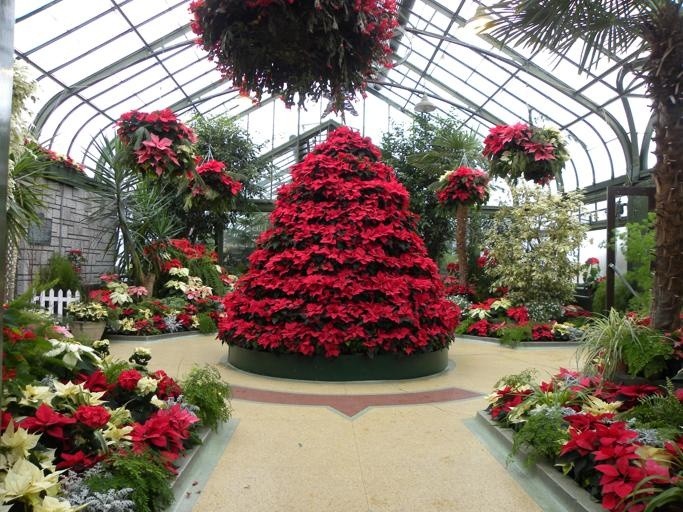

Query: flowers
(434, 167), (489, 214)
(109, 109), (206, 189)
(482, 123), (569, 189)
(191, 157), (243, 200)
(187, 1), (400, 119)
(64, 300), (109, 320)
(214, 125), (463, 360)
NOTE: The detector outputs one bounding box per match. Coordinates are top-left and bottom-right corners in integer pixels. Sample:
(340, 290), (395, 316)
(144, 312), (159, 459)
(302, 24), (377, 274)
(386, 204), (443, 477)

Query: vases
(65, 322), (106, 346)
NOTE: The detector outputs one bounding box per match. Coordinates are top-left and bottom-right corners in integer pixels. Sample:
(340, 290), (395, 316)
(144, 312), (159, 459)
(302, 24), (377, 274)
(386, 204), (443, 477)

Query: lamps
(464, 1), (494, 37)
